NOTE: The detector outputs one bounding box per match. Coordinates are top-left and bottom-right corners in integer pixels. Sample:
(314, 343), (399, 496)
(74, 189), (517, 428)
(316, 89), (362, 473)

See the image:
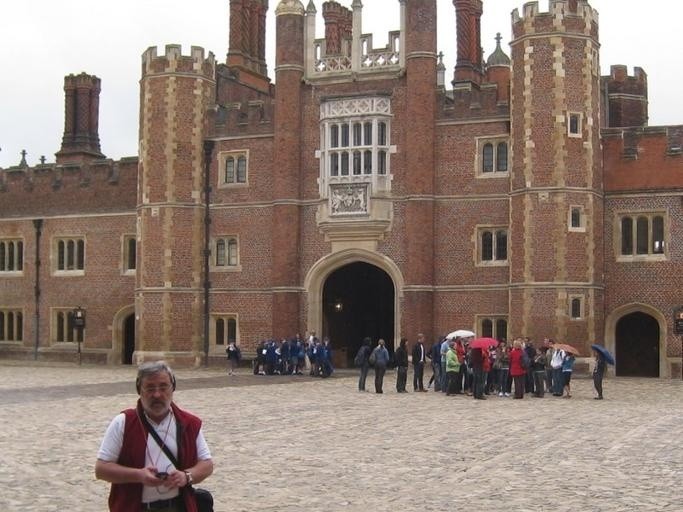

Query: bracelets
(181, 470), (192, 487)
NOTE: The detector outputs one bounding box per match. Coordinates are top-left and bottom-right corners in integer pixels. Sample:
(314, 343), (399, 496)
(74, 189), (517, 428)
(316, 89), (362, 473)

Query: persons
(257, 331), (334, 378)
(590, 343), (614, 365)
(396, 337), (409, 392)
(591, 352), (604, 400)
(372, 339), (390, 393)
(225, 340), (240, 375)
(411, 333), (429, 392)
(358, 337), (372, 393)
(425, 336), (576, 399)
(94, 359), (214, 511)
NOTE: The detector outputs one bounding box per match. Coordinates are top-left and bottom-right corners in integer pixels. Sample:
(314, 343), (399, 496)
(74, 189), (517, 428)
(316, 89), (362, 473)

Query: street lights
(72, 304), (84, 364)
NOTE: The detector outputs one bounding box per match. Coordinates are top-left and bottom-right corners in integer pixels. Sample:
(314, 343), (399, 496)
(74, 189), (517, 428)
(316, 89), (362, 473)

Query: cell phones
(156, 471), (168, 478)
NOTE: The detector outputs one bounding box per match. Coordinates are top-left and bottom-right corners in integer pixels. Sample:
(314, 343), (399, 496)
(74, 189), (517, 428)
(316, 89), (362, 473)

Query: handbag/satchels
(189, 487), (215, 512)
(369, 352), (376, 367)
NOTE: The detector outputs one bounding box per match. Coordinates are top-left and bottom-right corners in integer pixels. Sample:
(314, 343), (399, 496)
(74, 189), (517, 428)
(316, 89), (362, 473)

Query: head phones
(136, 361), (177, 393)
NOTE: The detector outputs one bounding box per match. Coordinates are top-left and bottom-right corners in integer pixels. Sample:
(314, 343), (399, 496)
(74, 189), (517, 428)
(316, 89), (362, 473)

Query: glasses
(140, 383), (177, 397)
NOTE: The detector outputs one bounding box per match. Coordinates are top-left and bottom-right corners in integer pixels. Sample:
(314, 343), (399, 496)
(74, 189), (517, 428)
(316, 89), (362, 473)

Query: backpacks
(352, 349), (365, 368)
(519, 353), (532, 371)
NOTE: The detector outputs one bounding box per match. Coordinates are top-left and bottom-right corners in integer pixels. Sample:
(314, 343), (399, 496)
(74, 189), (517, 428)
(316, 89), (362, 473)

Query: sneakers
(227, 368), (333, 378)
(356, 386), (604, 401)
